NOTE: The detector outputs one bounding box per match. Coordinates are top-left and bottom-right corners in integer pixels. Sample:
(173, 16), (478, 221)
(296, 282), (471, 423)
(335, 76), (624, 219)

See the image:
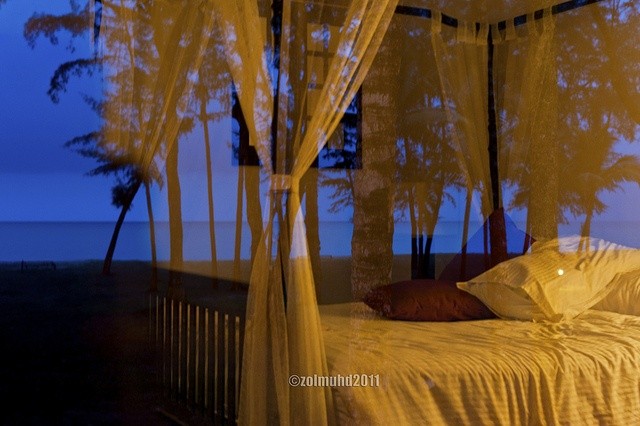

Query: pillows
(362, 278), (497, 321)
(456, 236), (639, 325)
(438, 207), (537, 284)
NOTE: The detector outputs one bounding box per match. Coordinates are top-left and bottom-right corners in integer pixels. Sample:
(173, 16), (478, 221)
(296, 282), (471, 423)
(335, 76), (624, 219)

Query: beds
(119, 1), (640, 426)
